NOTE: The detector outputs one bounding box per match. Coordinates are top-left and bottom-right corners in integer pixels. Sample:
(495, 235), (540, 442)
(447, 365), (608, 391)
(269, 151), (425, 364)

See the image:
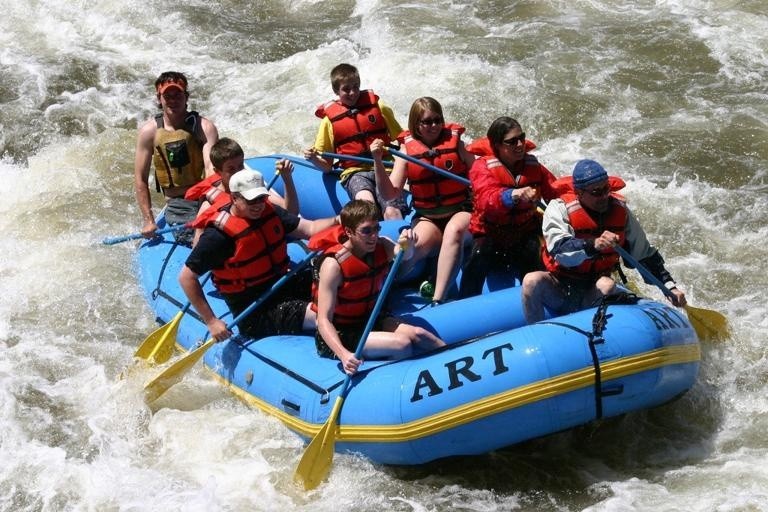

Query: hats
(573, 160), (607, 188)
(157, 78), (185, 95)
(229, 170), (270, 201)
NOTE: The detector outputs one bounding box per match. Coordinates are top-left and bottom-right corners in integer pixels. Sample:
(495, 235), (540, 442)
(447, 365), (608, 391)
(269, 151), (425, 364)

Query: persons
(310, 199), (446, 377)
(191, 135), (300, 249)
(369, 96), (476, 309)
(464, 116), (554, 312)
(177, 167), (342, 339)
(302, 62), (411, 221)
(134, 71), (343, 249)
(520, 159), (688, 324)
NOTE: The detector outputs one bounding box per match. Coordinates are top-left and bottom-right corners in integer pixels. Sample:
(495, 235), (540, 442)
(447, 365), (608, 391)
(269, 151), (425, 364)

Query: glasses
(421, 117), (443, 124)
(355, 225), (381, 234)
(582, 184), (609, 197)
(244, 197), (266, 204)
(502, 132), (525, 145)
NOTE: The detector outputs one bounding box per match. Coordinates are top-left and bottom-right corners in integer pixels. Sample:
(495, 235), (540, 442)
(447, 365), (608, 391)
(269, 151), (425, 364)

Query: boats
(138, 152), (702, 463)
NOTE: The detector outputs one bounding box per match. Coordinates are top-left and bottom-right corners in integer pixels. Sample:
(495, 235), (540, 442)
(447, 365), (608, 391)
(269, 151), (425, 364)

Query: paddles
(293, 239), (408, 493)
(612, 240), (734, 341)
(121, 169), (283, 379)
(140, 251), (320, 404)
